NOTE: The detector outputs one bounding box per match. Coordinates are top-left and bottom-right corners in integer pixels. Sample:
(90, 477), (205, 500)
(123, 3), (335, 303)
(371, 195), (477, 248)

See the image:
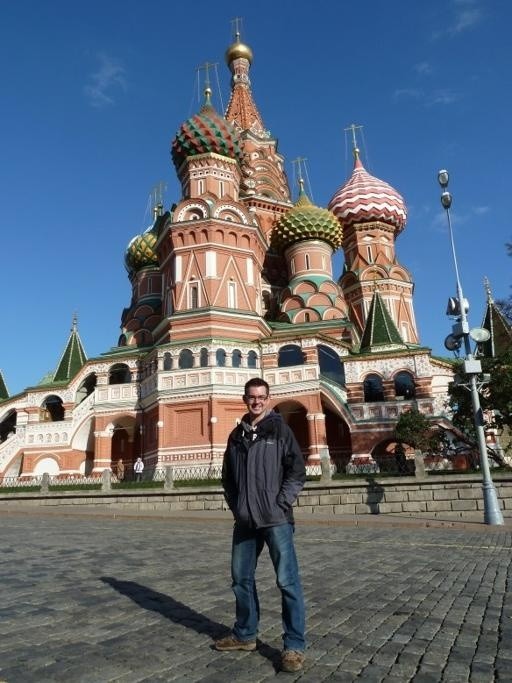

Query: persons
(116, 457), (125, 481)
(212, 376), (308, 669)
(133, 456), (144, 483)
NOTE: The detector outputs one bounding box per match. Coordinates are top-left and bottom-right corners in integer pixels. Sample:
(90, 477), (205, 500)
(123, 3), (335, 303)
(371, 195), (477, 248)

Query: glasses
(245, 395), (269, 403)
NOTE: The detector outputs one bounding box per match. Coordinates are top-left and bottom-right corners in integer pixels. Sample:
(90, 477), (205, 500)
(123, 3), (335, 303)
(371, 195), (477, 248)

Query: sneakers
(280, 648), (306, 672)
(215, 635), (256, 652)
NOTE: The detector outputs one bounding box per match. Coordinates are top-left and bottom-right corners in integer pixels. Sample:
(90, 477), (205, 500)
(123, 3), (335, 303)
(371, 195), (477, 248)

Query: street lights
(437, 169), (505, 527)
